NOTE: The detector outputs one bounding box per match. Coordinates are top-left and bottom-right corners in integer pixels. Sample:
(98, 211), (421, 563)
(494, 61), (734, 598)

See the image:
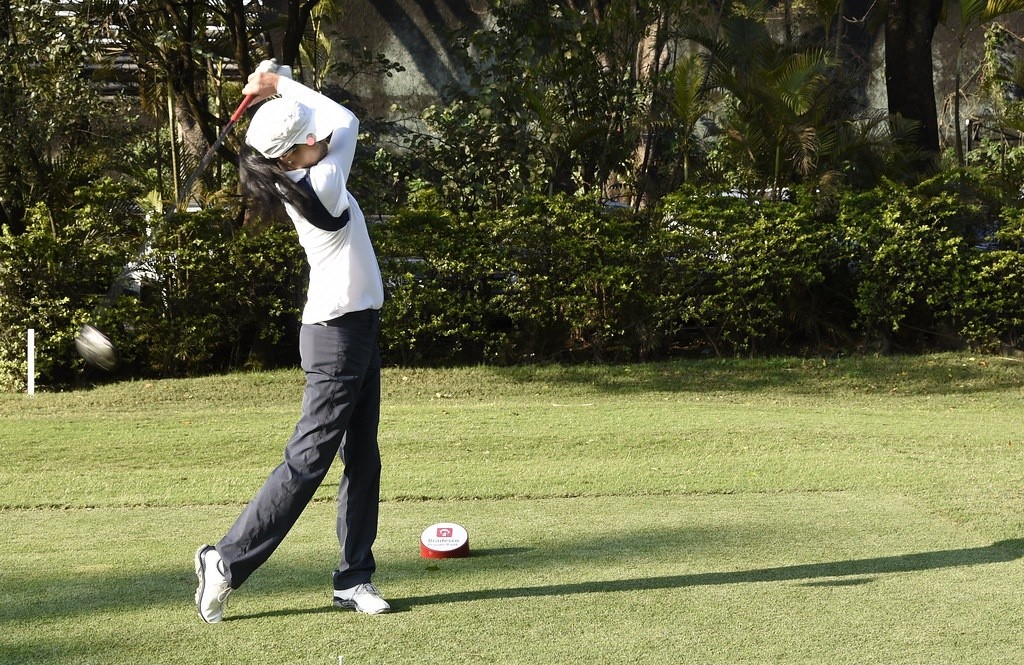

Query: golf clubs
(75, 56), (279, 373)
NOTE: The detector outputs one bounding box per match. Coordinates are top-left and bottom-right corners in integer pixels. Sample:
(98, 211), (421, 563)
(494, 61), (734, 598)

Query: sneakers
(332, 584), (391, 614)
(194, 545), (234, 624)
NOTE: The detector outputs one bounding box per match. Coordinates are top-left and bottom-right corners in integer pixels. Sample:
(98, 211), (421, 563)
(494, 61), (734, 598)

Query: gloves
(255, 58), (281, 74)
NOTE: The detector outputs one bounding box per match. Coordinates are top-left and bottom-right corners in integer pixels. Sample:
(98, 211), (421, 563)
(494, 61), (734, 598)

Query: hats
(245, 97), (334, 159)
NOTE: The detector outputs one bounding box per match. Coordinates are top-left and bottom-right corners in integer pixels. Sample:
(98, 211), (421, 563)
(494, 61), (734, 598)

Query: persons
(194, 59), (393, 626)
